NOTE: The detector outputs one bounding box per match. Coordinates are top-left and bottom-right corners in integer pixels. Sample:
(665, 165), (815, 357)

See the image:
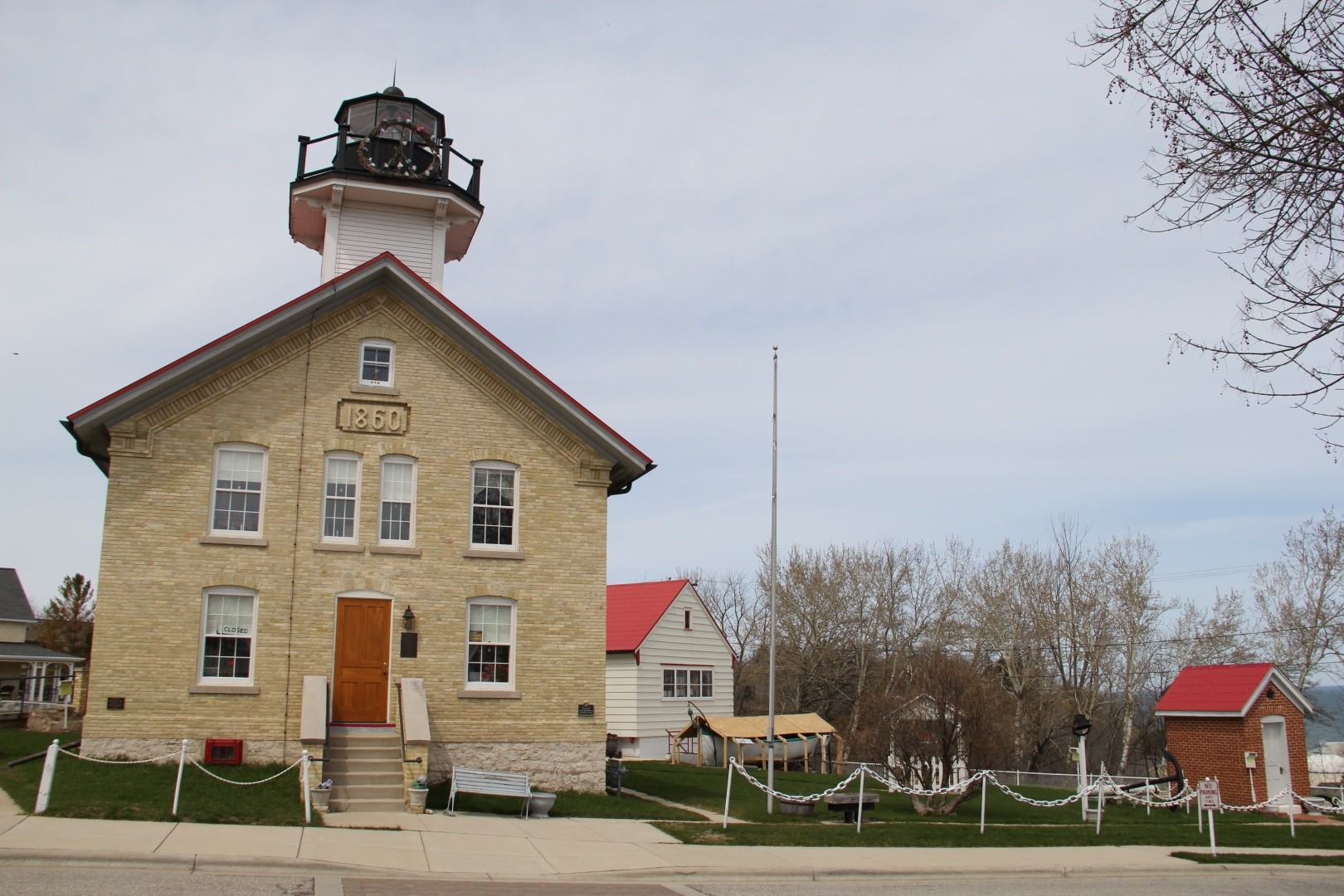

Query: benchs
(444, 766), (532, 819)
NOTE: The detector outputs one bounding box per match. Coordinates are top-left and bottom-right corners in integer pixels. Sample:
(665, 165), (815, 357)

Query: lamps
(402, 604), (415, 629)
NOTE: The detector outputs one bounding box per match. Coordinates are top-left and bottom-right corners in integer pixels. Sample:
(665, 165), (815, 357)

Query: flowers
(412, 775), (427, 790)
(316, 779), (333, 790)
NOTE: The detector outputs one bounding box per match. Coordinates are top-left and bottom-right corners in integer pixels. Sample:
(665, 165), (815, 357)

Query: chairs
(0, 684), (15, 699)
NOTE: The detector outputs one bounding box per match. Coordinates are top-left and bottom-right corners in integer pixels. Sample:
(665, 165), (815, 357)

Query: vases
(311, 787), (331, 805)
(408, 788), (429, 813)
(529, 792), (557, 817)
(1086, 808), (1104, 824)
(1298, 796), (1324, 815)
